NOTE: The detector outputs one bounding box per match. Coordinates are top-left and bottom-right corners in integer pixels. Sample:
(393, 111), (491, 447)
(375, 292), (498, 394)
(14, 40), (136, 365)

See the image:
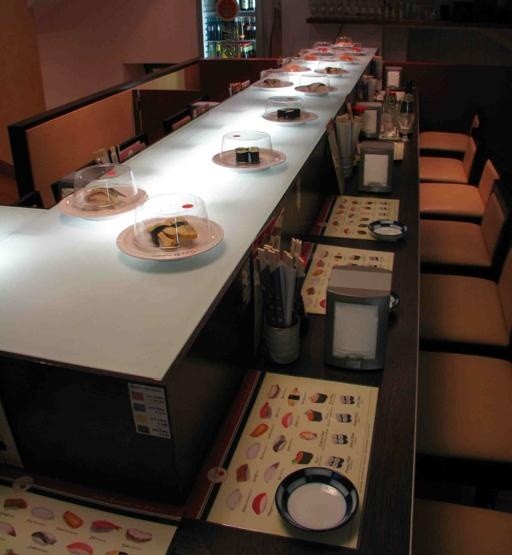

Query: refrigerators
(201, 0), (283, 58)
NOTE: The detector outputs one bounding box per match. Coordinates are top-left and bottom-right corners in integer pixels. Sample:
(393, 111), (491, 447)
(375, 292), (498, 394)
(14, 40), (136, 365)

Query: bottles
(209, 16), (256, 41)
(209, 41), (256, 58)
(236, 0), (256, 11)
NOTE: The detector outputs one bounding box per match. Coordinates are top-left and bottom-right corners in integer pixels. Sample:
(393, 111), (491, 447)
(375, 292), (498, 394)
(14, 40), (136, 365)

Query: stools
(413, 114), (511, 555)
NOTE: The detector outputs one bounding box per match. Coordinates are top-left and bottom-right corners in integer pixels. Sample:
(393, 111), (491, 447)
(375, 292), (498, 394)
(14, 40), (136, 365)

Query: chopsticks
(336, 113), (364, 162)
(257, 235), (303, 327)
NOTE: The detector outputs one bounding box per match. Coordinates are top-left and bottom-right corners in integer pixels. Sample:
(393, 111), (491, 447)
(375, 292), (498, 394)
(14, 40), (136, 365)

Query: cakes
(148, 215), (197, 250)
(277, 108), (300, 119)
(236, 147), (259, 164)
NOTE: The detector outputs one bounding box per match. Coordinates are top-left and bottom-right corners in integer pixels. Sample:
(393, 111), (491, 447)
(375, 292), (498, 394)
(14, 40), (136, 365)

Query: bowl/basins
(367, 219), (408, 241)
(274, 466), (360, 532)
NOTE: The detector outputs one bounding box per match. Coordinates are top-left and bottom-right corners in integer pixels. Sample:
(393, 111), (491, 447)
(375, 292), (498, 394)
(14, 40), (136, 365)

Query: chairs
(11, 105), (194, 210)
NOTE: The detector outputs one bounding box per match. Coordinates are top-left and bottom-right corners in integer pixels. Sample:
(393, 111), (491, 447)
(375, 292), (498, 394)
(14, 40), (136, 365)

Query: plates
(297, 44), (368, 64)
(59, 184), (149, 221)
(316, 69), (354, 76)
(117, 215), (224, 261)
(280, 66), (312, 75)
(212, 147), (286, 175)
(295, 85), (338, 96)
(253, 79), (294, 91)
(262, 109), (319, 127)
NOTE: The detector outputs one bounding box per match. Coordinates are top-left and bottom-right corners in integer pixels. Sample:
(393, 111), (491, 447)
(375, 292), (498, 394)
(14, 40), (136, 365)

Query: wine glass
(395, 113), (415, 143)
